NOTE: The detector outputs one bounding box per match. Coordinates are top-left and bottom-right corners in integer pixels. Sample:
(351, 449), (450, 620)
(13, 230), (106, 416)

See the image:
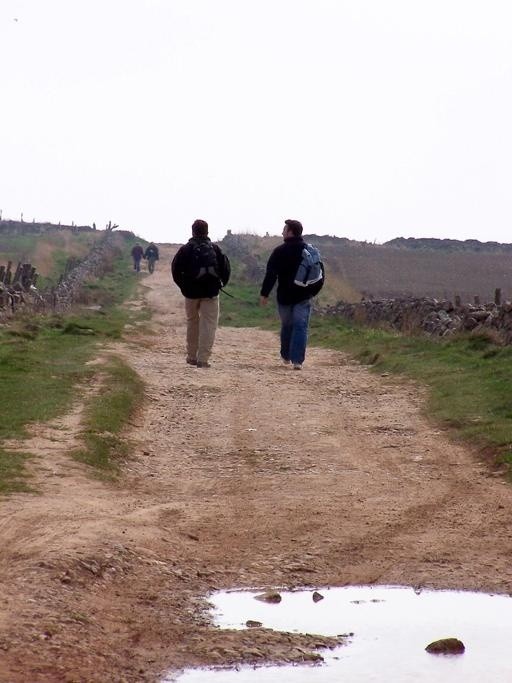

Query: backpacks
(292, 242), (324, 299)
(185, 242), (221, 298)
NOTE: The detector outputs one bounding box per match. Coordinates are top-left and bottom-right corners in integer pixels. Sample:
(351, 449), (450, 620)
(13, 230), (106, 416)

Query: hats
(192, 219), (208, 235)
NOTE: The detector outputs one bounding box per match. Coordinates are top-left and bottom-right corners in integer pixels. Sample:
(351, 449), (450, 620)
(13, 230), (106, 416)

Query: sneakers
(277, 357), (304, 370)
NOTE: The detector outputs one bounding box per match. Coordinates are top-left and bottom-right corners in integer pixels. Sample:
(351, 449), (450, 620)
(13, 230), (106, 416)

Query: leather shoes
(186, 356), (211, 368)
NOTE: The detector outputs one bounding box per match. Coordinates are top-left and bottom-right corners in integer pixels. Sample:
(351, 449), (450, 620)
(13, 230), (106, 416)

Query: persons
(171, 219), (231, 368)
(131, 241), (159, 274)
(258, 220), (325, 370)
